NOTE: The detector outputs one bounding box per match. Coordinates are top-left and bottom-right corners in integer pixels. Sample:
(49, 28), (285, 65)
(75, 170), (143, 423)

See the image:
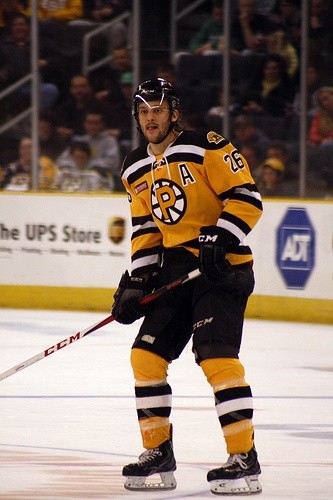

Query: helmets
(133, 77), (178, 118)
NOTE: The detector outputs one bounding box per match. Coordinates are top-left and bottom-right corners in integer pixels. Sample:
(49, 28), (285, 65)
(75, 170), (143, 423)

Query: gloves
(112, 271), (155, 325)
(197, 226), (240, 281)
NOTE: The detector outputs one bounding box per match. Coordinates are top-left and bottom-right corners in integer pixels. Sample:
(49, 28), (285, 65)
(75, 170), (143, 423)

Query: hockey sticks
(0, 268), (202, 382)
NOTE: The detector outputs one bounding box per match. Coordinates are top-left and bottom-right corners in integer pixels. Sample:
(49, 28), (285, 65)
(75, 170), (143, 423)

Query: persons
(110, 78), (263, 495)
(0, 0), (333, 200)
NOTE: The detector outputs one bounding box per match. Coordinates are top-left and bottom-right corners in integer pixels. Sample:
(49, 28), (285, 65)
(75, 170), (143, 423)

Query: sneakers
(207, 444), (261, 494)
(121, 423), (177, 489)
